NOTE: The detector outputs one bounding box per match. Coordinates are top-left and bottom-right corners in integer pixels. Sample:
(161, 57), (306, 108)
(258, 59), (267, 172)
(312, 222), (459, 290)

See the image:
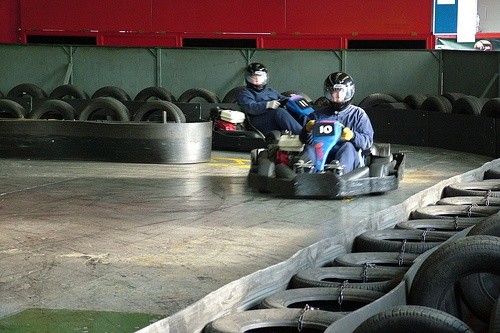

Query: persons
(236, 62), (309, 134)
(300, 72), (374, 174)
